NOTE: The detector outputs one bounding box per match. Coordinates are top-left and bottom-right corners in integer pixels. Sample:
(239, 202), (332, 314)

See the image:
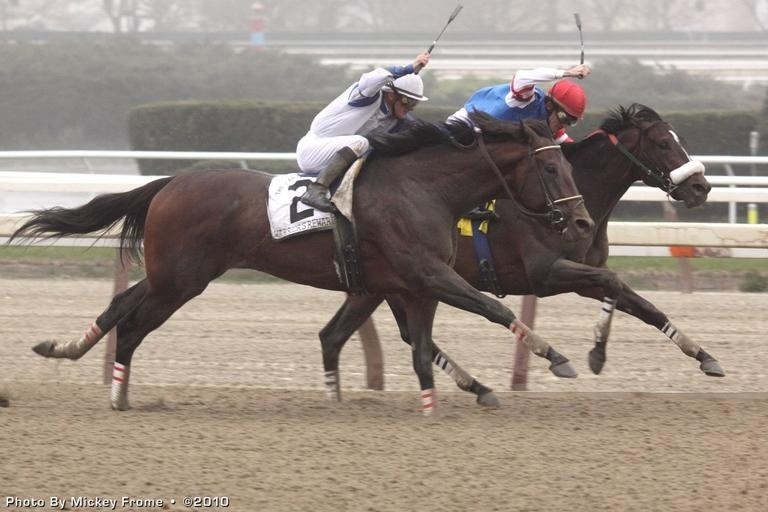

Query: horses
(319, 102), (725, 407)
(0, 104), (595, 416)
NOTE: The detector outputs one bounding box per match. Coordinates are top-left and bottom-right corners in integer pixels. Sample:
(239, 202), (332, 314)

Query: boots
(301, 146), (356, 213)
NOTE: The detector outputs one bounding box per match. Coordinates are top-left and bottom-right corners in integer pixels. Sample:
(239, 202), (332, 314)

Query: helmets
(548, 80), (586, 118)
(382, 73), (429, 102)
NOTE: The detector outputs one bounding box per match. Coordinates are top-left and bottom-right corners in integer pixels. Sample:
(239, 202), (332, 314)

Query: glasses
(398, 94), (417, 106)
(556, 110), (577, 125)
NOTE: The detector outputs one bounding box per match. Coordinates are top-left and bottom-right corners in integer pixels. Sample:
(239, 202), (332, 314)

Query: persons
(296, 52), (431, 214)
(445, 63), (592, 296)
